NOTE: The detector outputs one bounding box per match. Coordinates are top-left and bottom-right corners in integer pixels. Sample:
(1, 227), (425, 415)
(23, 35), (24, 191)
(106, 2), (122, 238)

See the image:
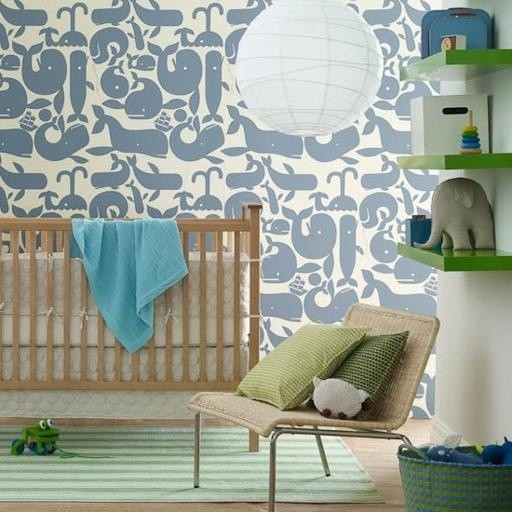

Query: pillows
(234, 321), (410, 421)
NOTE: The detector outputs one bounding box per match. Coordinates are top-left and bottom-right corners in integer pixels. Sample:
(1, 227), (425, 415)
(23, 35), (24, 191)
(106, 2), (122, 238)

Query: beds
(1, 204), (265, 451)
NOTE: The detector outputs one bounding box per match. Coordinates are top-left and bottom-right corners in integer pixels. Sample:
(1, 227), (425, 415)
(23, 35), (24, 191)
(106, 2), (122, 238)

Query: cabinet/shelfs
(394, 49), (510, 274)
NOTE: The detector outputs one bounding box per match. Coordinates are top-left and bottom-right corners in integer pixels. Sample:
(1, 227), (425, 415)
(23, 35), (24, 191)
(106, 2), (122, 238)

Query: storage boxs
(408, 93), (490, 157)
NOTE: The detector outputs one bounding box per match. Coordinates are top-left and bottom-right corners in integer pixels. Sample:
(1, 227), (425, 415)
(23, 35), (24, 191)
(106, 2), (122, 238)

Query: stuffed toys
(308, 377), (373, 421)
(424, 436), (512, 465)
(11, 418), (60, 456)
(413, 177), (495, 251)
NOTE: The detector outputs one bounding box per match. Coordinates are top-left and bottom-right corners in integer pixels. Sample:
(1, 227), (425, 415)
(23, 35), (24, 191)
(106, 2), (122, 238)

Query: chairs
(187, 302), (440, 512)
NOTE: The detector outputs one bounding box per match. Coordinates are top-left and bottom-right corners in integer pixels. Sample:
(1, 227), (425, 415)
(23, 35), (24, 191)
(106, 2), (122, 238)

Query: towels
(66, 216), (189, 356)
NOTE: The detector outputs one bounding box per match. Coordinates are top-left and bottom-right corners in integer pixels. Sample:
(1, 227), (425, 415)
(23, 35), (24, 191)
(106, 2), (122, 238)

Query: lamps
(234, 1), (386, 139)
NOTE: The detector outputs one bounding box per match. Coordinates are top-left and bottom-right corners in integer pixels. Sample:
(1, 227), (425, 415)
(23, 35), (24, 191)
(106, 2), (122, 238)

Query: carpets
(1, 425), (387, 506)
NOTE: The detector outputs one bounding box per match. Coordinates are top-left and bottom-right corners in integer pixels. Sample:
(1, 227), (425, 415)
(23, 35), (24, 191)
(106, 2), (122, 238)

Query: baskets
(395, 435), (512, 512)
